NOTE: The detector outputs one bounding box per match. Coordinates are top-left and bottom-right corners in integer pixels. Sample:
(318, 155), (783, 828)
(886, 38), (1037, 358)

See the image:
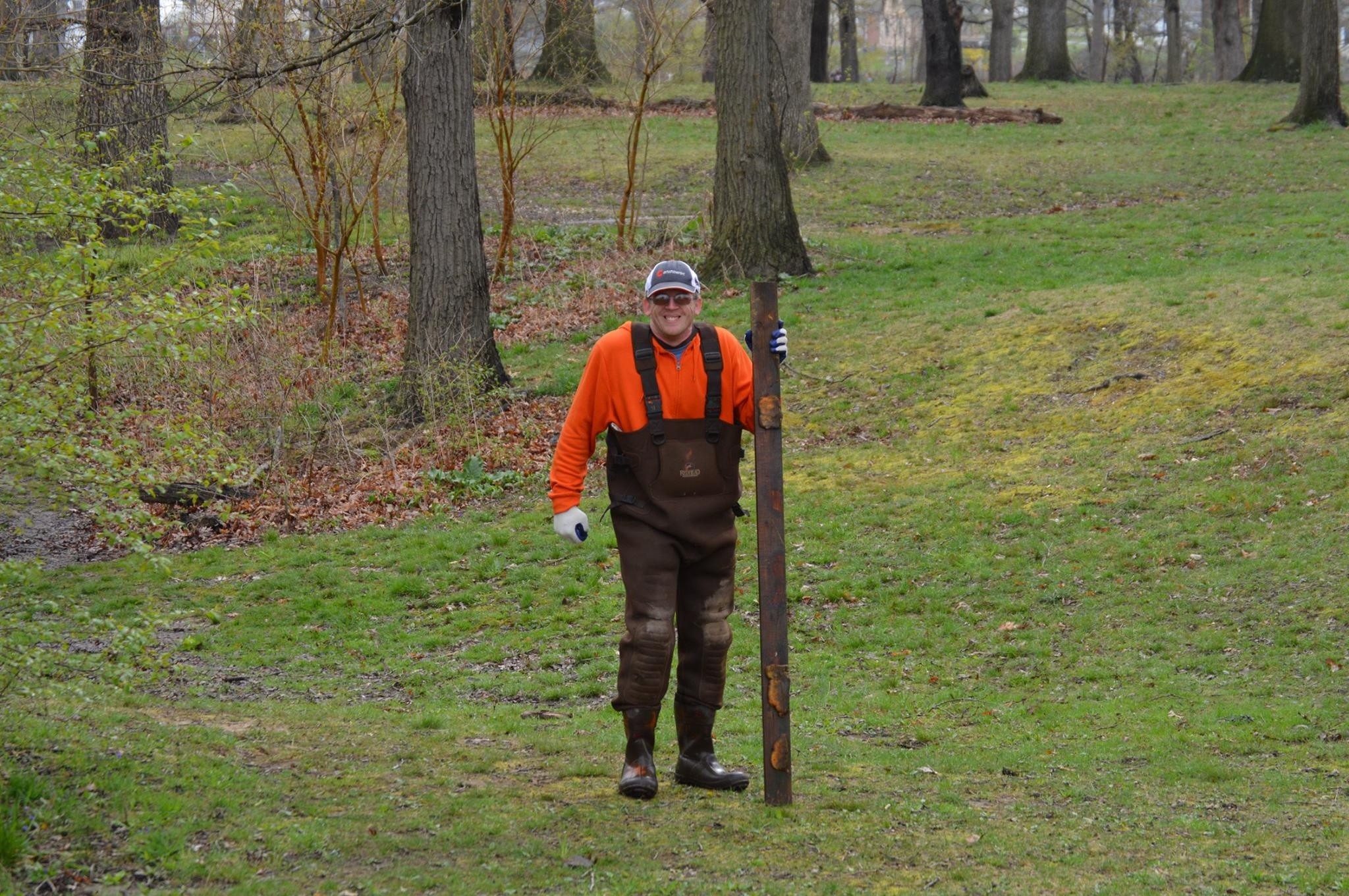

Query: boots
(620, 705), (662, 801)
(674, 694), (748, 792)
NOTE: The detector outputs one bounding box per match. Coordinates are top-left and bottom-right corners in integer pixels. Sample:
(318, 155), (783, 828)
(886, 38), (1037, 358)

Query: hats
(645, 260), (700, 298)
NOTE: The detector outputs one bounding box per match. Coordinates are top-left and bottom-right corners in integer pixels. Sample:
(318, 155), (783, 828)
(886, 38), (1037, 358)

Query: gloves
(553, 505), (589, 544)
(744, 320), (788, 365)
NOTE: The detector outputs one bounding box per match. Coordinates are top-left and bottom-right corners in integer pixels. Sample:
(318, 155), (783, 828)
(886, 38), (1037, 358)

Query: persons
(547, 261), (789, 796)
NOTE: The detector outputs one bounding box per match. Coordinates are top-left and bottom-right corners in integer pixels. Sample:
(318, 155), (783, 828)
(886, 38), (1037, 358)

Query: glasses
(648, 294), (697, 305)
